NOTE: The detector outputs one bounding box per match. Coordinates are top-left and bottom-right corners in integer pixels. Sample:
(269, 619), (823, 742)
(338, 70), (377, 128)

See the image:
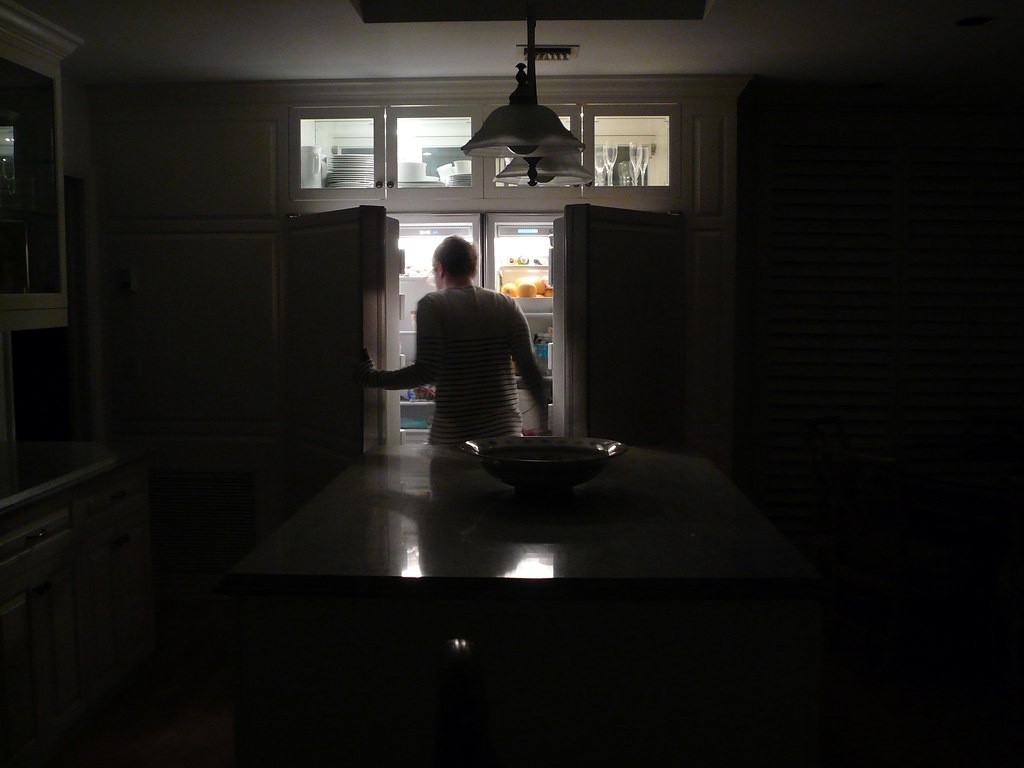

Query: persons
(349, 235), (549, 444)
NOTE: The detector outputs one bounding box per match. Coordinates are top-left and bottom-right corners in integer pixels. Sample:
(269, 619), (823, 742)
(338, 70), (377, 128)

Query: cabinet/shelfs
(0, 0), (86, 331)
(0, 438), (160, 768)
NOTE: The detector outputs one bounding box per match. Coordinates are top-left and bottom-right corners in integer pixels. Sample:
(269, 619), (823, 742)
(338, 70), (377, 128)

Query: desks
(829, 451), (1024, 709)
(208, 435), (828, 768)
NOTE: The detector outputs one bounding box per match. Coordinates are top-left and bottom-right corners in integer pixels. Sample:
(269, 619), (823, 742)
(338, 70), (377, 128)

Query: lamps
(460, 0), (594, 188)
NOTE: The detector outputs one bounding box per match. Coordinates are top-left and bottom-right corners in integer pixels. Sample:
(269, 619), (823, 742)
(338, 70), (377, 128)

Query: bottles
(533, 333), (548, 360)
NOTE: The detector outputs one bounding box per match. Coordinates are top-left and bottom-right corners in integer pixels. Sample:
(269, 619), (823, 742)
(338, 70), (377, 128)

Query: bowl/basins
(459, 435), (629, 494)
(398, 162), (427, 181)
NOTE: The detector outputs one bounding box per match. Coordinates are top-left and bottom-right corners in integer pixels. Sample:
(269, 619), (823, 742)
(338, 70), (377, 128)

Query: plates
(325, 154), (374, 187)
(398, 181), (446, 188)
(449, 174), (472, 186)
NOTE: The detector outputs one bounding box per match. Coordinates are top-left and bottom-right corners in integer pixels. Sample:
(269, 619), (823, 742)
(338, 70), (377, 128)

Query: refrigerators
(287, 206), (688, 450)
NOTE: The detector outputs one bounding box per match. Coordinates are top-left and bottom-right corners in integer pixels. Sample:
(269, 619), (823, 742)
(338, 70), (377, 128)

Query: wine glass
(594, 144), (617, 186)
(618, 141), (651, 186)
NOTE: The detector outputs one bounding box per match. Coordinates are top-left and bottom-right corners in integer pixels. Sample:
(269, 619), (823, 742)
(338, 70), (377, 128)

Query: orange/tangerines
(500, 278), (554, 299)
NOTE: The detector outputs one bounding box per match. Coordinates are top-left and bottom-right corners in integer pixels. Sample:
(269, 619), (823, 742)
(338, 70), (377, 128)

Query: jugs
(301, 145), (322, 188)
